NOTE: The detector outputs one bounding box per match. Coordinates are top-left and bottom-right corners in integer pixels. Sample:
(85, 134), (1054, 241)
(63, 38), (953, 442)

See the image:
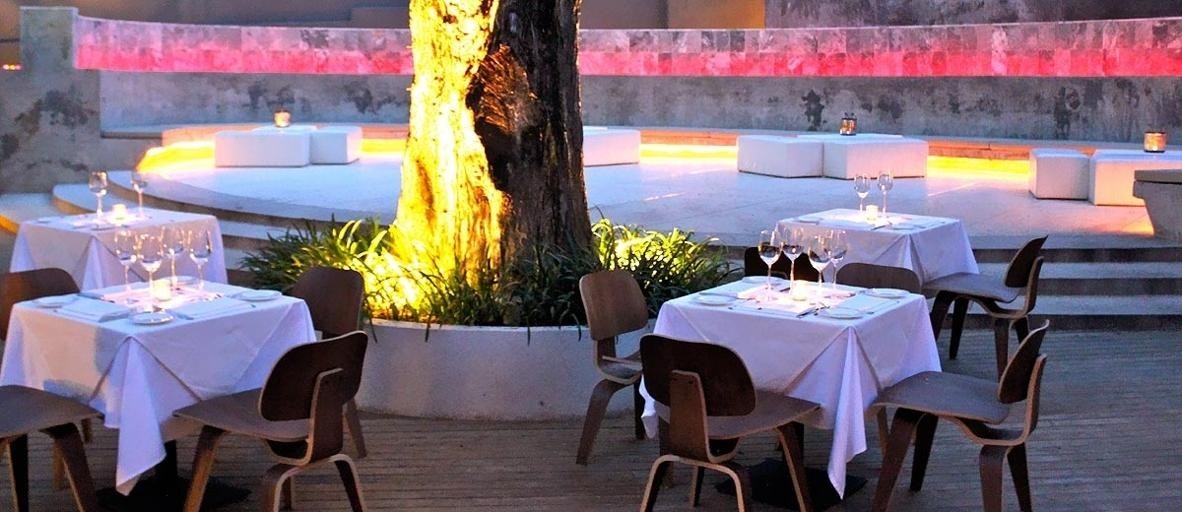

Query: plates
(128, 311), (175, 324)
(860, 288), (912, 298)
(818, 307), (863, 319)
(231, 290), (283, 302)
(31, 295), (81, 308)
(689, 292), (737, 304)
(742, 276), (777, 283)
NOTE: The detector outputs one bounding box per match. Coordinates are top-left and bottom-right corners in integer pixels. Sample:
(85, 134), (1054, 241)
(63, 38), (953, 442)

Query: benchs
(215, 126), (364, 169)
(583, 126), (639, 166)
(1028, 149), (1182, 207)
(737, 132), (928, 181)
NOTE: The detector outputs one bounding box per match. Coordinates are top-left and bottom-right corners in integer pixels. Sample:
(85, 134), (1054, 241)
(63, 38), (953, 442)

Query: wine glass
(852, 171), (894, 220)
(757, 221), (848, 304)
(89, 170), (107, 224)
(129, 165), (150, 220)
(112, 224), (213, 307)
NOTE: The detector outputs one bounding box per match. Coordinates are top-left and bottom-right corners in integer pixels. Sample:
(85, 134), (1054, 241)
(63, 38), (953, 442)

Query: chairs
(928, 237), (1047, 384)
(0, 385), (103, 511)
(837, 263), (921, 296)
(293, 268), (369, 460)
(172, 326), (365, 512)
(578, 272), (650, 464)
(873, 321), (1049, 512)
(2, 269), (84, 334)
(743, 246), (825, 283)
(640, 335), (821, 512)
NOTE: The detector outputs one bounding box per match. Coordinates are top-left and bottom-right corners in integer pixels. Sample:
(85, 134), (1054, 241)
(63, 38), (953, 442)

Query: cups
(839, 118), (856, 136)
(111, 202), (126, 222)
(1143, 132), (1166, 153)
(274, 110), (291, 128)
